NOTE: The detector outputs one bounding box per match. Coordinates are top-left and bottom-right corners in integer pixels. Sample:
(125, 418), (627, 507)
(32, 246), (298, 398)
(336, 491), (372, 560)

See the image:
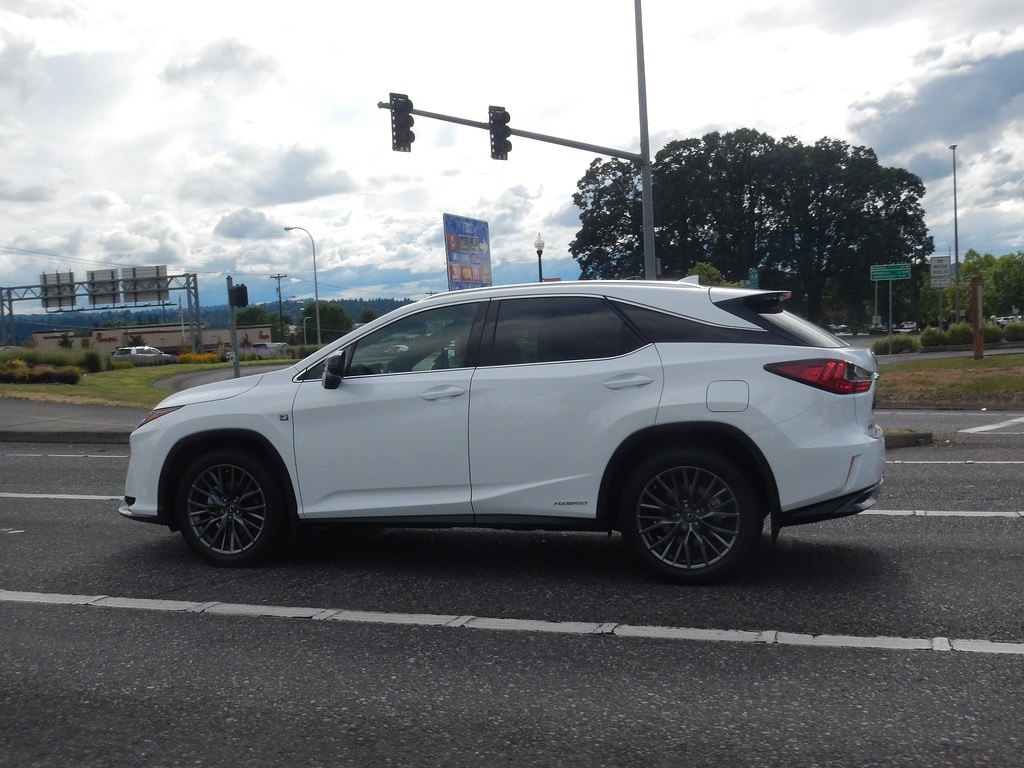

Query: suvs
(115, 345), (169, 356)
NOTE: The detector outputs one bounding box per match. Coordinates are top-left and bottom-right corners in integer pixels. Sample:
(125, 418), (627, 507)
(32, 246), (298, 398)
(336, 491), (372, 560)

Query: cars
(395, 345), (408, 352)
(997, 316), (1010, 327)
(117, 282), (891, 590)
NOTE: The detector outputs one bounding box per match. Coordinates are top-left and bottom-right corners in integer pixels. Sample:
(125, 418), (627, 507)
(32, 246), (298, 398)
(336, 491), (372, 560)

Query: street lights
(304, 317), (311, 345)
(949, 145), (961, 325)
(534, 233), (545, 283)
(284, 226), (321, 345)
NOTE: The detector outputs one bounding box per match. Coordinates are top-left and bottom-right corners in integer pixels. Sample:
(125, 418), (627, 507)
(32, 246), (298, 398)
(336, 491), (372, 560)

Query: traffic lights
(389, 93), (415, 152)
(489, 106), (512, 160)
